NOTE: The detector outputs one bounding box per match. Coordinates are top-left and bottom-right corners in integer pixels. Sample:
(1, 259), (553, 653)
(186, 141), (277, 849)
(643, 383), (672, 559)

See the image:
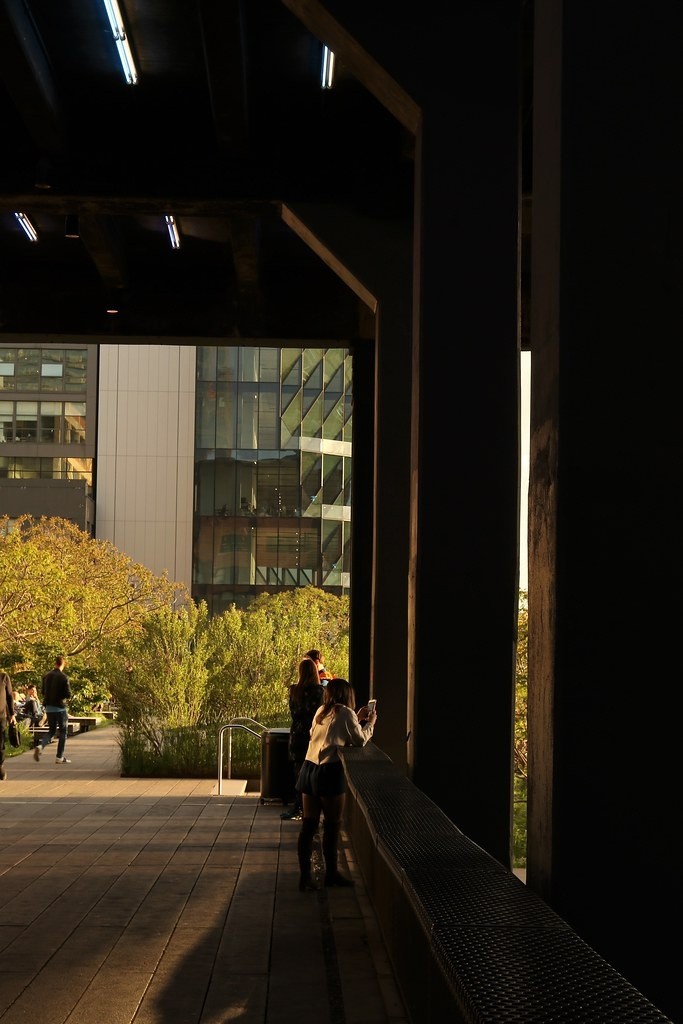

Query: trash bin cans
(259, 727), (295, 807)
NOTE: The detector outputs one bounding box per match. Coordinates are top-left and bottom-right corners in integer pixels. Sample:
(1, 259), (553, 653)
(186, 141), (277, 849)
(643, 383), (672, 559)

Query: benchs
(25, 712), (113, 746)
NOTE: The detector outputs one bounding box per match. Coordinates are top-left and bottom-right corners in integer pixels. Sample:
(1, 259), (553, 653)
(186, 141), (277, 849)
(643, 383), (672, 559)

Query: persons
(23, 685), (52, 743)
(34, 655), (71, 764)
(286, 648), (333, 820)
(296, 678), (378, 894)
(0, 671), (18, 780)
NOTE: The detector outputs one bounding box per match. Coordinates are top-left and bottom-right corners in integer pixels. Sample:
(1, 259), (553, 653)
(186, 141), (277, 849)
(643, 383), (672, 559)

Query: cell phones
(366, 699), (377, 721)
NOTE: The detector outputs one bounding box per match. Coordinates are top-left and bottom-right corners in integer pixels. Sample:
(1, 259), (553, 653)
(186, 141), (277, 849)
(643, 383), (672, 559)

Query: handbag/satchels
(8, 719), (20, 748)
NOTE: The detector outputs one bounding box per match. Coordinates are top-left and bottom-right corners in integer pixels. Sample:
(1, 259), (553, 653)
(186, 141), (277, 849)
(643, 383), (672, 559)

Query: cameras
(320, 679), (331, 687)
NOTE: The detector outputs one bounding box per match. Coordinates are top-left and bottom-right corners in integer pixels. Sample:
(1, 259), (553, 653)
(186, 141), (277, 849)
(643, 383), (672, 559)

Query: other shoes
(299, 876), (320, 892)
(34, 745), (42, 762)
(323, 870), (355, 887)
(56, 757), (72, 764)
(280, 805), (301, 820)
